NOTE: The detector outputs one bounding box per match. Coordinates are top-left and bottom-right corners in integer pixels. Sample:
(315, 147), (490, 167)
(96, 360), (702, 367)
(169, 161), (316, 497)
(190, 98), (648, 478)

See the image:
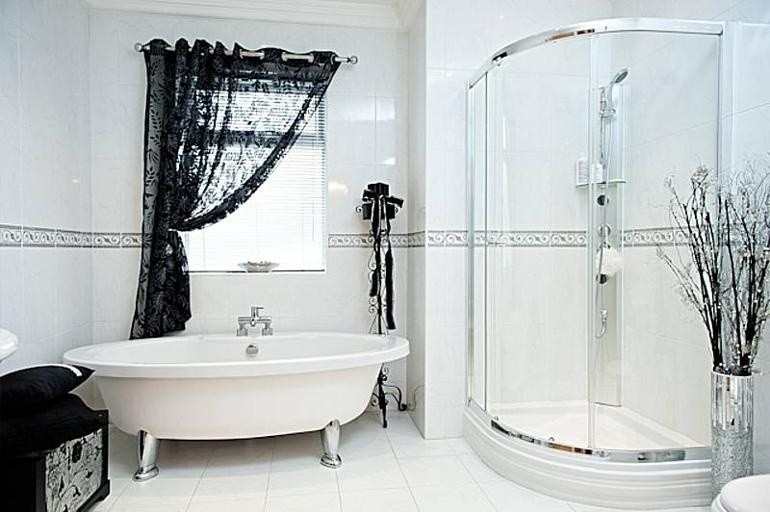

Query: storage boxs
(0, 407), (111, 512)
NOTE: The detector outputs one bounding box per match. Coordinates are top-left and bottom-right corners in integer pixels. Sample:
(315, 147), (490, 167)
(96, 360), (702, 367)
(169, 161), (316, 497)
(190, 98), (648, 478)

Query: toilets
(709, 474), (770, 512)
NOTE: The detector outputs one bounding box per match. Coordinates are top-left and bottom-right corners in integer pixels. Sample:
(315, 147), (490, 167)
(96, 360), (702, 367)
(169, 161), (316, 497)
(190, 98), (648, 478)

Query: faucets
(248, 307), (263, 327)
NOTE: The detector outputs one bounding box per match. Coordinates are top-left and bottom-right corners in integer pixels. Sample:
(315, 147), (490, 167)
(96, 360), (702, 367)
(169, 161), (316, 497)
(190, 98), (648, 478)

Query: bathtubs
(65, 334), (410, 480)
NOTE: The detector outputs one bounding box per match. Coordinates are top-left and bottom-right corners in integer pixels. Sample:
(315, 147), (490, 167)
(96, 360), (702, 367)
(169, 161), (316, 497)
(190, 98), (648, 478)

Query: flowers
(654, 155), (770, 378)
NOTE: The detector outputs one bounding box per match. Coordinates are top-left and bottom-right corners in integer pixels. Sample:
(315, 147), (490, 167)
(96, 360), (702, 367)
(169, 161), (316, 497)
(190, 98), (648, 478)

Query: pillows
(0, 364), (96, 402)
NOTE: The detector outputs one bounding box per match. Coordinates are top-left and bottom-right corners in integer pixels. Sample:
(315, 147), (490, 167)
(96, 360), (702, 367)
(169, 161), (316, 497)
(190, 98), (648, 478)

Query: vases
(709, 366), (754, 502)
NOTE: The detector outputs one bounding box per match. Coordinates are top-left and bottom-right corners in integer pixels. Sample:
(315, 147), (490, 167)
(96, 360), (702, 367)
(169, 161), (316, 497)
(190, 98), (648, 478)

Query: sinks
(0, 328), (18, 362)
